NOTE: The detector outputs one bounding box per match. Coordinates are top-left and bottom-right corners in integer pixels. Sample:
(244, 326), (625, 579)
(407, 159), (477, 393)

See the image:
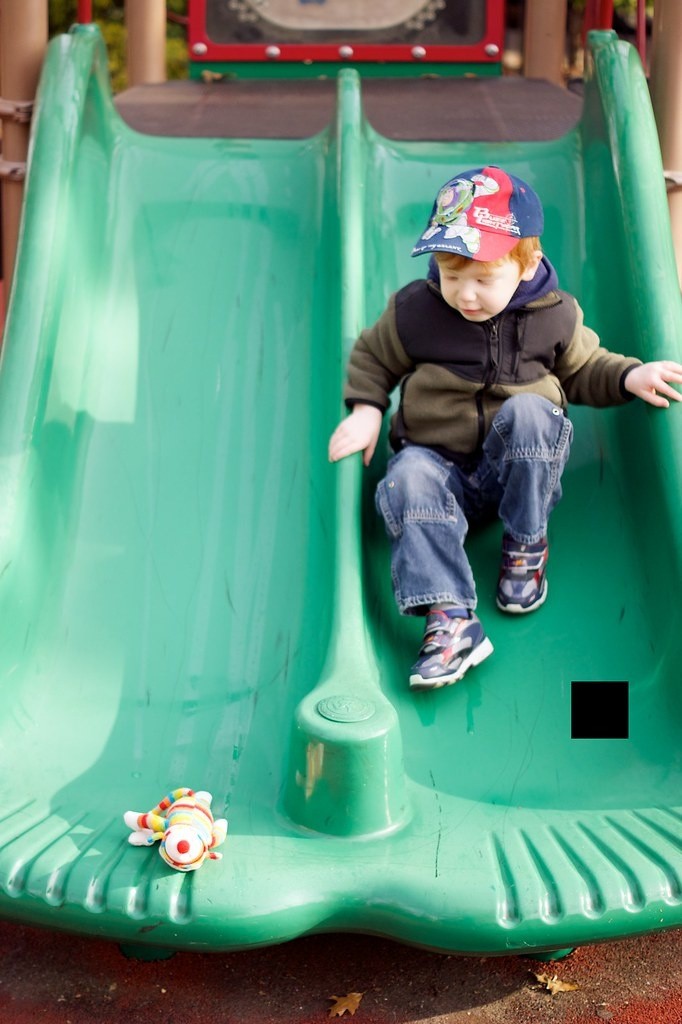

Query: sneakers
(496, 531), (548, 613)
(408, 609), (493, 688)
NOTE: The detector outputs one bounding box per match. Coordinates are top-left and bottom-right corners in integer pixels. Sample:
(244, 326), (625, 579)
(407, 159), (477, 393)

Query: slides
(0, 18), (680, 963)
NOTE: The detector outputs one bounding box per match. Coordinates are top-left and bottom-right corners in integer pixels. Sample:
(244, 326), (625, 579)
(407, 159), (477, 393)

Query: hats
(411, 166), (543, 261)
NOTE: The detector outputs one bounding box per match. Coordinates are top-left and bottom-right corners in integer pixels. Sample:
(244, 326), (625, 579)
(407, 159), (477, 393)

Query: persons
(327, 168), (682, 686)
